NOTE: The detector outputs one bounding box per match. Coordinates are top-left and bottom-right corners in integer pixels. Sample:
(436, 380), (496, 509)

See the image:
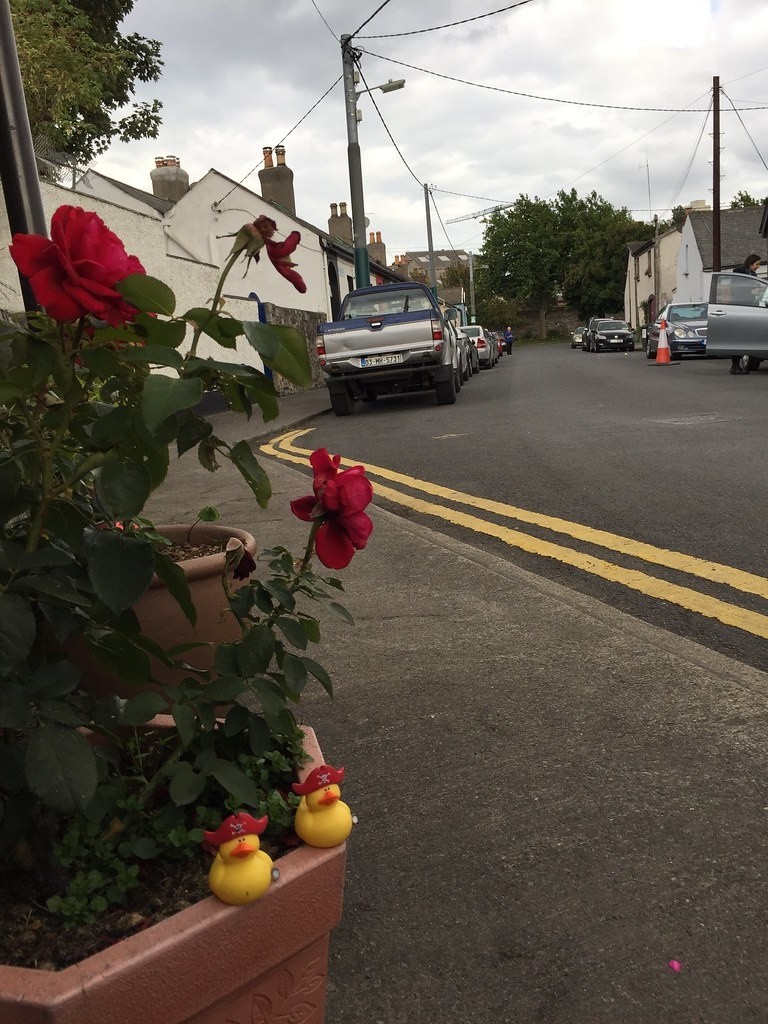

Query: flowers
(1, 203), (373, 926)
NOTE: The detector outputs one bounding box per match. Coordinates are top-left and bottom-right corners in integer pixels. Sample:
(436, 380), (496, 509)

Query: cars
(452, 324), (507, 384)
(645, 301), (709, 358)
(705, 271), (768, 370)
(569, 326), (586, 349)
(589, 319), (635, 353)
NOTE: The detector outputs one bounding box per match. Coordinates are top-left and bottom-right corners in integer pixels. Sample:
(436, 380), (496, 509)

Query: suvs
(582, 316), (616, 351)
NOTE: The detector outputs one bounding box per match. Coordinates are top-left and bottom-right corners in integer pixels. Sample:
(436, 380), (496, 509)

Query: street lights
(340, 76), (407, 290)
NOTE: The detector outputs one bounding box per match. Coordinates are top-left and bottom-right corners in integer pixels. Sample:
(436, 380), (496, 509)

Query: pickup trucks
(316, 282), (461, 417)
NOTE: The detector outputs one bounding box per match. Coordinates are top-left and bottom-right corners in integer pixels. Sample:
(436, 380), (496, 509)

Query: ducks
(292, 764), (353, 848)
(205, 812), (277, 907)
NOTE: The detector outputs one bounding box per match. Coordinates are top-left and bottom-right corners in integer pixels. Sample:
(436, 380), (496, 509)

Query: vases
(62, 520), (256, 717)
(0, 711), (347, 1024)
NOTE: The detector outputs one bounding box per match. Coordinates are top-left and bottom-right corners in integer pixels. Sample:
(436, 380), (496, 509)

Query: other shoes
(730, 364), (750, 375)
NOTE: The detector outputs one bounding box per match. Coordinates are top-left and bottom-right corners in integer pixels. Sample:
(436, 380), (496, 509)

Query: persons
(504, 326), (515, 356)
(729, 255), (764, 375)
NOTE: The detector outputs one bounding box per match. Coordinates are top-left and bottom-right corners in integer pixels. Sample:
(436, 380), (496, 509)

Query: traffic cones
(647, 319), (681, 365)
